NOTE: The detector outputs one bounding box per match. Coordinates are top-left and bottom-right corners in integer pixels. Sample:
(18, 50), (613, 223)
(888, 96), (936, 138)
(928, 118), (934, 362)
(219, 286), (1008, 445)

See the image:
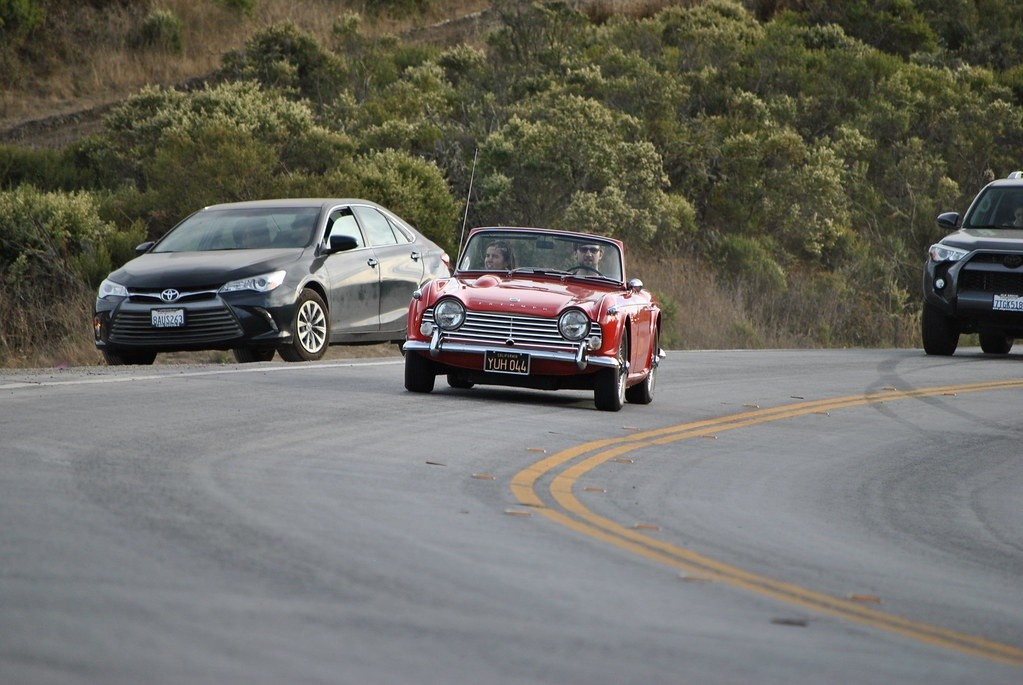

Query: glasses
(577, 247), (600, 254)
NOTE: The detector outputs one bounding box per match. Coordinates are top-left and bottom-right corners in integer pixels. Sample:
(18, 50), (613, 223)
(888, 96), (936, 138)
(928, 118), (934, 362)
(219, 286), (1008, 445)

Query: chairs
(232, 217), (267, 248)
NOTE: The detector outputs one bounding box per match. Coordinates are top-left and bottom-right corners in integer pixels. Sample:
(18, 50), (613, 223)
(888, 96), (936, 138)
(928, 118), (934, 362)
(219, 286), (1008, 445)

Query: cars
(399, 224), (667, 410)
(91, 198), (453, 363)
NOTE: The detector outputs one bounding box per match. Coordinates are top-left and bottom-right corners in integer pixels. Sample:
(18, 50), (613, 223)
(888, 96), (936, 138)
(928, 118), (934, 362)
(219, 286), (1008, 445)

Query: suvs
(920, 171), (1023, 357)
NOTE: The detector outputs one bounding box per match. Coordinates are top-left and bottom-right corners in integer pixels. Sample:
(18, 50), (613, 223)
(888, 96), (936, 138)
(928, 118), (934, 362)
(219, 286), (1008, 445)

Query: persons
(484, 240), (515, 270)
(573, 242), (604, 276)
(241, 225), (261, 248)
(1001, 204), (1023, 226)
(289, 214), (314, 240)
